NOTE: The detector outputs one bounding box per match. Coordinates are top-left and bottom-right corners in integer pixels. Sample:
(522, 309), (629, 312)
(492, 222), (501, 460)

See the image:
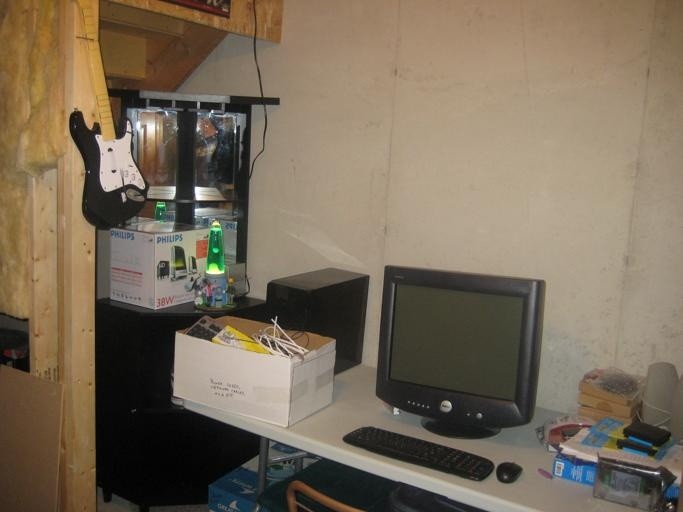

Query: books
(551, 415), (682, 502)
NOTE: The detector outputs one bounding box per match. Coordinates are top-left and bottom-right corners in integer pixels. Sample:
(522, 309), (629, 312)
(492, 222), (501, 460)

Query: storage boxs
(205, 442), (318, 510)
(105, 224), (214, 309)
(170, 314), (337, 427)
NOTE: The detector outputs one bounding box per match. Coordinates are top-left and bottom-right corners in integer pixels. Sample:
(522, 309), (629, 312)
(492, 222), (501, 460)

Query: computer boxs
(169, 245), (187, 280)
(265, 267), (370, 375)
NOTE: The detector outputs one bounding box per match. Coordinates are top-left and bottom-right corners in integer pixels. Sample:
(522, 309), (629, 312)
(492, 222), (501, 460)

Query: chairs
(285, 480), (361, 510)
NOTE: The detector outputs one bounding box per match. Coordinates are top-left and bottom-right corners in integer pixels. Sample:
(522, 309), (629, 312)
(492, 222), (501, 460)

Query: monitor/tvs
(375, 264), (547, 440)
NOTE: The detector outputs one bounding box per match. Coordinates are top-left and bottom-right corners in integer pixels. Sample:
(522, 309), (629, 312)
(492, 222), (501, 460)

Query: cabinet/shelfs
(96, 295), (269, 511)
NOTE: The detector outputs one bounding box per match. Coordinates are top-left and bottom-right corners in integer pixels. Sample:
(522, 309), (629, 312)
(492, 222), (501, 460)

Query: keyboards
(342, 426), (494, 482)
(184, 315), (224, 342)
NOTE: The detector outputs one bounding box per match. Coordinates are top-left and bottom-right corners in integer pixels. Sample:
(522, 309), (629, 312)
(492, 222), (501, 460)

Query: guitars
(69, 1), (148, 229)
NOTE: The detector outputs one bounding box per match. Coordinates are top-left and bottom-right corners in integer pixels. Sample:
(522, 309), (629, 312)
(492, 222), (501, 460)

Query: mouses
(495, 461), (523, 483)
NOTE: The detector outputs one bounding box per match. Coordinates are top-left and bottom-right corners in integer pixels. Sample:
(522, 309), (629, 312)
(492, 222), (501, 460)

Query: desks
(167, 357), (681, 511)
